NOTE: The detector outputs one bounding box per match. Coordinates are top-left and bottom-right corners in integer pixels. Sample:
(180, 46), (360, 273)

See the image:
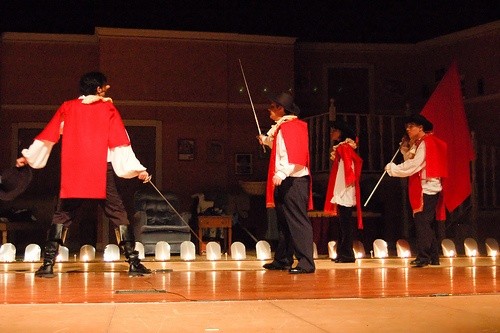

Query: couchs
(131, 190), (193, 254)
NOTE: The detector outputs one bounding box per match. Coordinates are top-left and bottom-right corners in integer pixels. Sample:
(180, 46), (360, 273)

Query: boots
(115, 223), (150, 275)
(35, 224), (69, 277)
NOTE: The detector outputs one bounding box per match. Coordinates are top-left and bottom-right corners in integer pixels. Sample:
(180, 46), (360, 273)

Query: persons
(382, 112), (448, 265)
(256, 92), (315, 274)
(15, 72), (153, 277)
(327, 118), (364, 262)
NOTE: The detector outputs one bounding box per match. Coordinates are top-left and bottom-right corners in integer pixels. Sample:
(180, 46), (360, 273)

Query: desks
(199, 215), (232, 255)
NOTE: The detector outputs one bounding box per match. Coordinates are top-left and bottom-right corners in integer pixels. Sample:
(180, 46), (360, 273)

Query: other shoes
(334, 258), (348, 263)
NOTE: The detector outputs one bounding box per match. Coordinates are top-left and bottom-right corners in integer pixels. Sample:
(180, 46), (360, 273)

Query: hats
(400, 113), (433, 131)
(269, 91), (300, 115)
(329, 120), (355, 137)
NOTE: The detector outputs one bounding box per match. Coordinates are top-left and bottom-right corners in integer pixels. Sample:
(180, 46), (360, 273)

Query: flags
(398, 61), (475, 221)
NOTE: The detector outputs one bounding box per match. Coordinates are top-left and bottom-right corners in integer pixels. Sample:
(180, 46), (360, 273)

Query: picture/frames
(177, 137), (195, 161)
(234, 152), (254, 175)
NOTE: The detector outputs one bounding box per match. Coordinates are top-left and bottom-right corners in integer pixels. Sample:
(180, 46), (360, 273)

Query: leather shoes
(409, 258), (439, 265)
(288, 267), (306, 273)
(263, 263), (284, 270)
